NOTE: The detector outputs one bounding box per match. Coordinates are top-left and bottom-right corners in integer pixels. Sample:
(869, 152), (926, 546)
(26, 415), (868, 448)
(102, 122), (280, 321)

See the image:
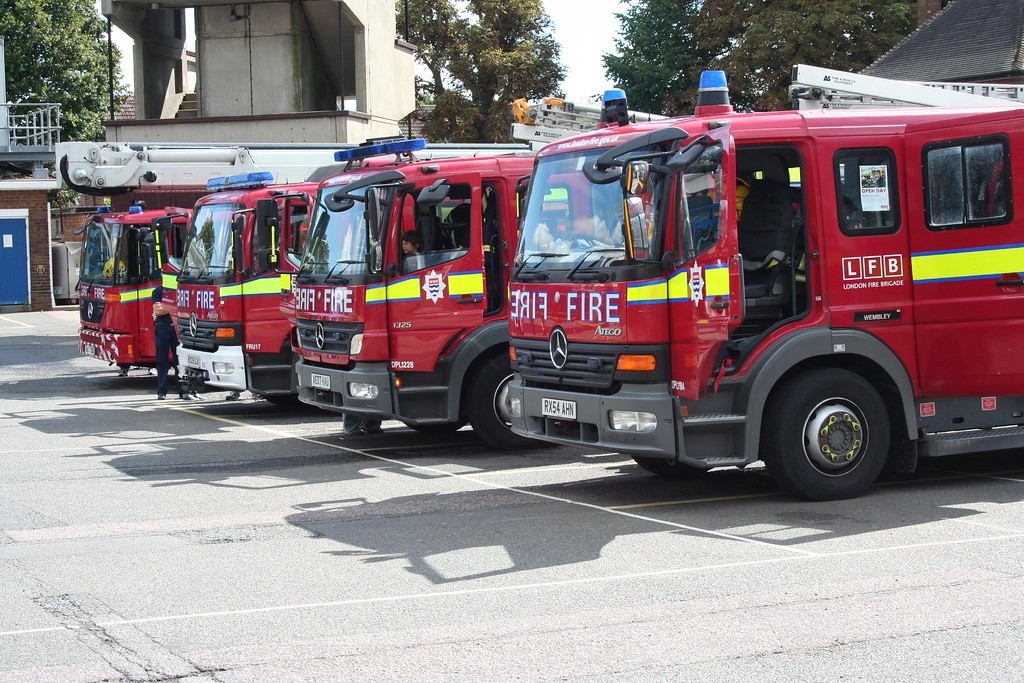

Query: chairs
(412, 215), (444, 263)
(739, 185), (805, 325)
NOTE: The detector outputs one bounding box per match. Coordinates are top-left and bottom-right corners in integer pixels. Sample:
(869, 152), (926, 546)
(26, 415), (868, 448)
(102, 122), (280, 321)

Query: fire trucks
(167, 156), (343, 413)
(291, 93), (682, 449)
(51, 140), (536, 383)
(505, 63), (1024, 502)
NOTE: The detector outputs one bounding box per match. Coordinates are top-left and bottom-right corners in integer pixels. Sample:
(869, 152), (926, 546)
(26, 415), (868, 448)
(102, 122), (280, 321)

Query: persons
(871, 168), (887, 187)
(400, 231), (425, 258)
(339, 412), (386, 436)
(150, 267), (198, 402)
